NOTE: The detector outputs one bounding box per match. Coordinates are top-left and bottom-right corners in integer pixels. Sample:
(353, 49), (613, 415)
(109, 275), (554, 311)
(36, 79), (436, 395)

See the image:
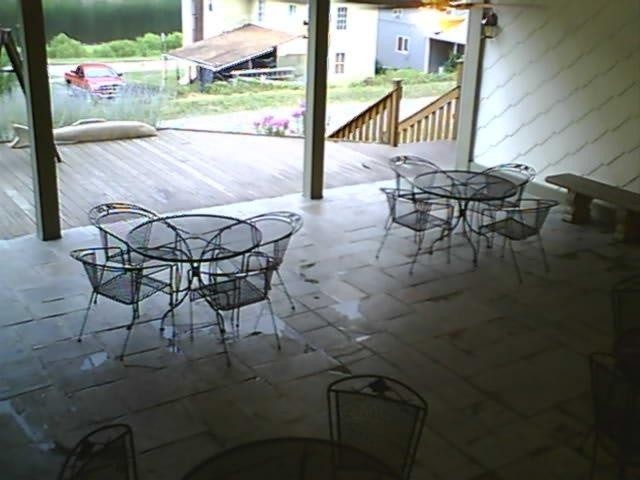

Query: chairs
(610, 273), (640, 369)
(374, 155), (560, 284)
(70, 246), (176, 362)
(187, 253), (283, 368)
(88, 201), (194, 319)
(326, 373), (429, 480)
(576, 352), (640, 480)
(197, 211), (304, 328)
(55, 422), (138, 480)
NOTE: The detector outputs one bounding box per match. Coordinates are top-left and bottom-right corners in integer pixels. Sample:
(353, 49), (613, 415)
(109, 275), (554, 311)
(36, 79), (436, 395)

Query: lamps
(481, 13), (498, 38)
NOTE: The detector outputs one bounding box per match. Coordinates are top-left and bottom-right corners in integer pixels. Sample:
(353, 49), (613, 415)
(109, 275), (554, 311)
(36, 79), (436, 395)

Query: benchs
(545, 173), (640, 244)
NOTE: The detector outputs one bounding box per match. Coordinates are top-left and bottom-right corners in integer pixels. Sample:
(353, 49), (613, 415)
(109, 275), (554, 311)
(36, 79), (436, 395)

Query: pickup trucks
(65, 63), (126, 101)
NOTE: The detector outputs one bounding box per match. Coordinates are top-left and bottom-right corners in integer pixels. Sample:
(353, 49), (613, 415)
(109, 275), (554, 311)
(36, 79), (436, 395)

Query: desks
(180, 436), (403, 479)
(126, 214), (263, 332)
